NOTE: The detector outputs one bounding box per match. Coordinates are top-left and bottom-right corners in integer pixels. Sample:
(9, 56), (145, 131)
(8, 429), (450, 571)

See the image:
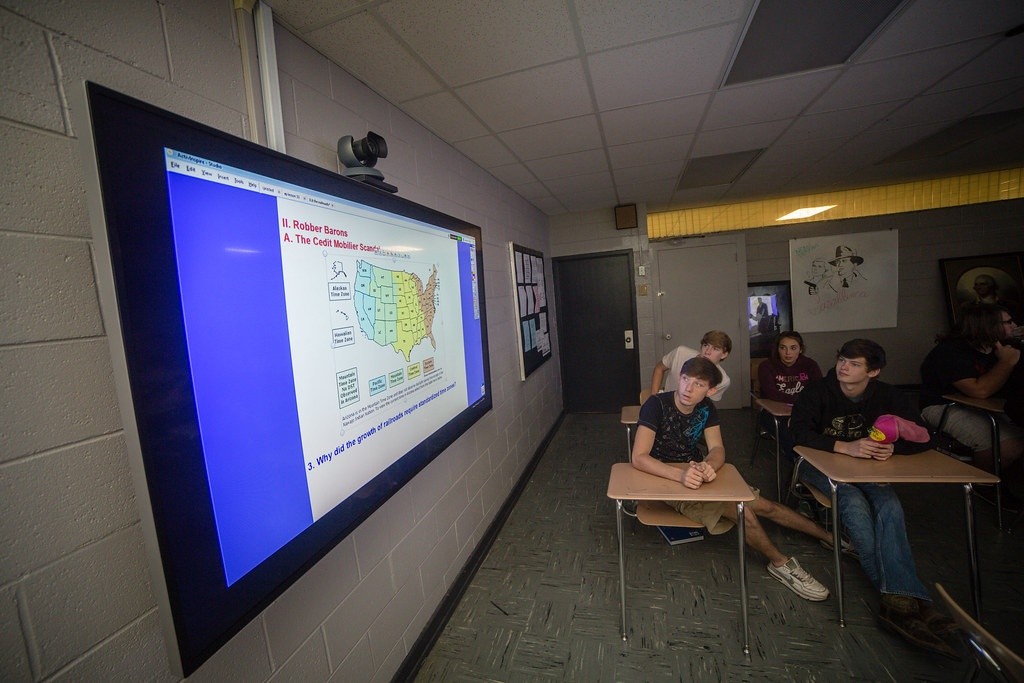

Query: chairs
(622, 500), (704, 528)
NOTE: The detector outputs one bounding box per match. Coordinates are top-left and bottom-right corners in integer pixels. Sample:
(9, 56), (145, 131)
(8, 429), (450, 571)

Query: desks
(621, 406), (641, 463)
(936, 393), (1006, 530)
(606, 462), (754, 656)
(750, 399), (793, 503)
(789, 446), (1002, 627)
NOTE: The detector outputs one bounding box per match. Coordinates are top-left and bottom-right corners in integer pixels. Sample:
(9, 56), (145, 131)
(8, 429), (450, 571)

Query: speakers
(615, 204), (638, 230)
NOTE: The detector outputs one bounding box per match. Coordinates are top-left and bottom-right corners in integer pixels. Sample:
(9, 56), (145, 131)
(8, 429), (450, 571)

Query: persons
(916, 302), (1024, 540)
(650, 329), (732, 402)
(788, 338), (965, 663)
(749, 297), (768, 326)
(630, 357), (862, 603)
(757, 330), (841, 541)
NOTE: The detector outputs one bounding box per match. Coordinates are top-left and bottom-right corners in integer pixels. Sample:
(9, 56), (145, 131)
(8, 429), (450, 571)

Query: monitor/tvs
(86, 79), (493, 679)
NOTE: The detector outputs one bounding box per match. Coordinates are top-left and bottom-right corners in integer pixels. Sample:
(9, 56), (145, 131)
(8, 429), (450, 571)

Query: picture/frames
(747, 279), (793, 358)
(939, 250), (1024, 327)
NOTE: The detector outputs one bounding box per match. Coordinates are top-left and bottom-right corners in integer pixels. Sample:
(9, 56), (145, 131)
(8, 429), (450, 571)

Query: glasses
(1001, 319), (1012, 324)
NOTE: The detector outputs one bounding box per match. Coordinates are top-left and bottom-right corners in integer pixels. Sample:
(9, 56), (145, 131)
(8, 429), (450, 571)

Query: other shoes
(919, 606), (961, 637)
(800, 503), (816, 522)
(819, 508), (832, 525)
(882, 612), (967, 662)
(972, 484), (1024, 514)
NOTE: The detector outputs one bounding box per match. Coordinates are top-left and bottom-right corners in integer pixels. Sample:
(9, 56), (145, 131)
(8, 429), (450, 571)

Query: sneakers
(820, 532), (861, 559)
(767, 557), (829, 601)
